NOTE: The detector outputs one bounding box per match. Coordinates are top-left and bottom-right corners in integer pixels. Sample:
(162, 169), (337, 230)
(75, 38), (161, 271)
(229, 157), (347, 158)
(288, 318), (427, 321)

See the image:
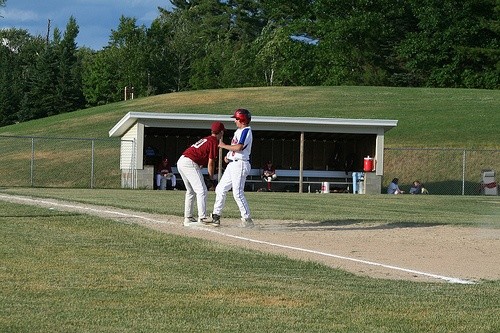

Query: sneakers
(240, 218), (254, 228)
(204, 219), (220, 226)
(198, 218), (215, 225)
(184, 217), (198, 226)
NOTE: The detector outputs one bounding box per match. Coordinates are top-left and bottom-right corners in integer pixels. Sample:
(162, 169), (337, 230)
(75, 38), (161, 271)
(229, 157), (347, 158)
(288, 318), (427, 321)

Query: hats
(162, 158), (168, 162)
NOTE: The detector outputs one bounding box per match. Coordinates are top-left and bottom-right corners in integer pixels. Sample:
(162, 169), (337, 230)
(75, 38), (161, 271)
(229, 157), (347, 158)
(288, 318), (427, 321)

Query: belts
(229, 160), (249, 162)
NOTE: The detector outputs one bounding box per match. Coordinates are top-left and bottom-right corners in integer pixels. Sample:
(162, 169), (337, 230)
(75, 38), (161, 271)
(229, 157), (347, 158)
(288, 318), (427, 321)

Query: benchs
(156, 167), (353, 195)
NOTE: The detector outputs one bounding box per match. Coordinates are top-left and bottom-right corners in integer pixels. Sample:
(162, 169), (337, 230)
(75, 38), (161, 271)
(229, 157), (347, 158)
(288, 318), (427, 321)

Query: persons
(412, 180), (429, 194)
(261, 161), (277, 192)
(156, 157), (178, 191)
(208, 155), (226, 191)
(202, 108), (254, 227)
(176, 120), (226, 225)
(387, 178), (405, 194)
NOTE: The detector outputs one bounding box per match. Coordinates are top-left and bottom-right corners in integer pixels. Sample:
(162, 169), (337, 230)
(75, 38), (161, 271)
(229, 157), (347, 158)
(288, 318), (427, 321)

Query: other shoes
(157, 187), (160, 190)
(171, 187), (178, 190)
(267, 189), (272, 192)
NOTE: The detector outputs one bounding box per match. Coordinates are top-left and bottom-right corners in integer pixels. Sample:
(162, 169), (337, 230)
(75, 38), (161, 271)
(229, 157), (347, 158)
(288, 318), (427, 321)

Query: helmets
(230, 109), (251, 124)
(211, 122), (224, 135)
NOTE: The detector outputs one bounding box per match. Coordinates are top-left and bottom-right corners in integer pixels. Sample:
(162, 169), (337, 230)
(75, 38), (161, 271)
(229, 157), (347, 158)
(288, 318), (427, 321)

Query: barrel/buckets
(321, 182), (329, 193)
